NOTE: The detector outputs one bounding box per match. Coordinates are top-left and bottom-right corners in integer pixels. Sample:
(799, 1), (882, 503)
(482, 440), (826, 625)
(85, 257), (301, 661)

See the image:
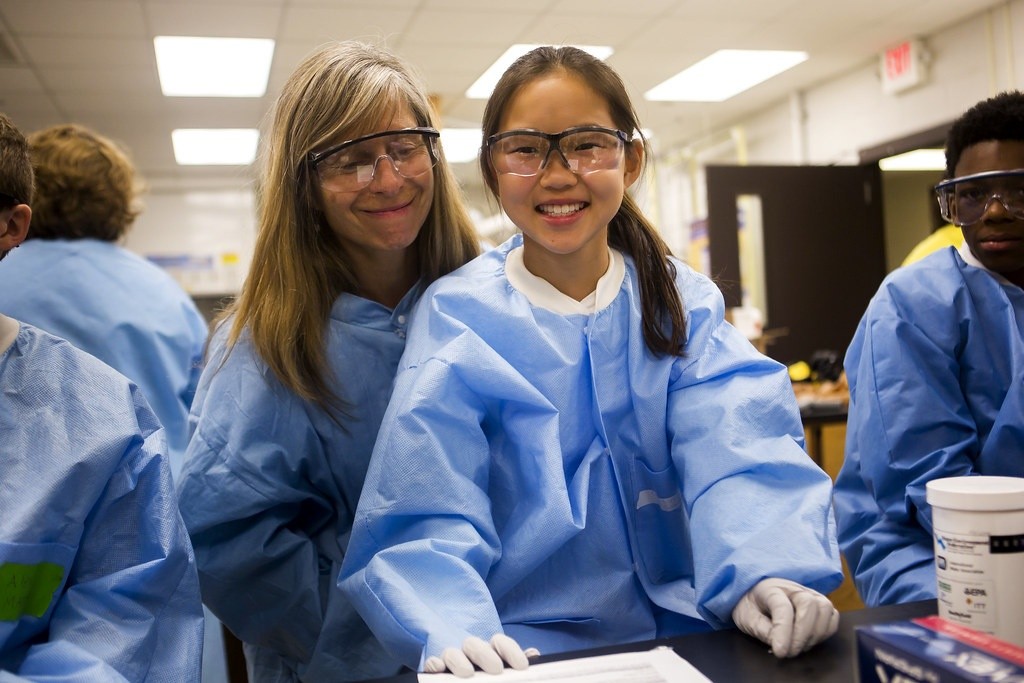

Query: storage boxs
(855, 616), (1024, 683)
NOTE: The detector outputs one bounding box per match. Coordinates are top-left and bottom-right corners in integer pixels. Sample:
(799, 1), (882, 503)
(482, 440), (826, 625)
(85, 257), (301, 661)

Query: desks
(374, 598), (939, 683)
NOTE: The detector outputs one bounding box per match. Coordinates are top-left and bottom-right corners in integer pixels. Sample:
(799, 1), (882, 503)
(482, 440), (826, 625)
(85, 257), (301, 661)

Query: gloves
(423, 633), (540, 677)
(732, 577), (839, 657)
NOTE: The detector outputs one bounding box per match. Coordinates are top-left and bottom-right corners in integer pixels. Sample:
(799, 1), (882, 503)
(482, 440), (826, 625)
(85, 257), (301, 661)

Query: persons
(0, 113), (205, 683)
(177, 41), (495, 683)
(829, 91), (1024, 623)
(302, 45), (845, 682)
(0, 123), (210, 486)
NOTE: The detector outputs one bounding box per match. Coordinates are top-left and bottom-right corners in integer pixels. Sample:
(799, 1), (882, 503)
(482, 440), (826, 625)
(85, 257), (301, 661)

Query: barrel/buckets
(925, 475), (1024, 650)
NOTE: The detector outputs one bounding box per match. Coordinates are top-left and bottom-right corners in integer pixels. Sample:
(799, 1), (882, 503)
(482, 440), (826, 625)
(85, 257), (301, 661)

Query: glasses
(935, 168), (1024, 226)
(486, 125), (633, 177)
(304, 126), (441, 192)
